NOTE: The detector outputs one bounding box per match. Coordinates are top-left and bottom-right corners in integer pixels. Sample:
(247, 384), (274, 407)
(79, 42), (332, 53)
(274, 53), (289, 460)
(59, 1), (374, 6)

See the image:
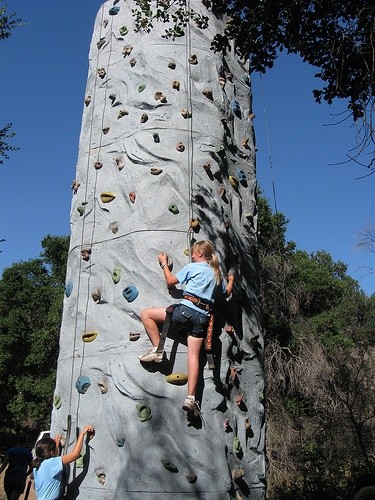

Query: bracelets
(160, 261), (167, 268)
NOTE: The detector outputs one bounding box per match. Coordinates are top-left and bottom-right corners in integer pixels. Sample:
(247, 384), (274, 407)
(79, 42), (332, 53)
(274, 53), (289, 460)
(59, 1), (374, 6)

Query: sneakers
(138, 346), (166, 362)
(182, 395), (201, 421)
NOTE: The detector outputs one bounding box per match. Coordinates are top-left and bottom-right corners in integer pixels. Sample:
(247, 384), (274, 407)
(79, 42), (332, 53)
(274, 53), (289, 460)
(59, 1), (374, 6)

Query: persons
(0, 432), (33, 500)
(33, 425), (94, 500)
(137, 240), (235, 422)
